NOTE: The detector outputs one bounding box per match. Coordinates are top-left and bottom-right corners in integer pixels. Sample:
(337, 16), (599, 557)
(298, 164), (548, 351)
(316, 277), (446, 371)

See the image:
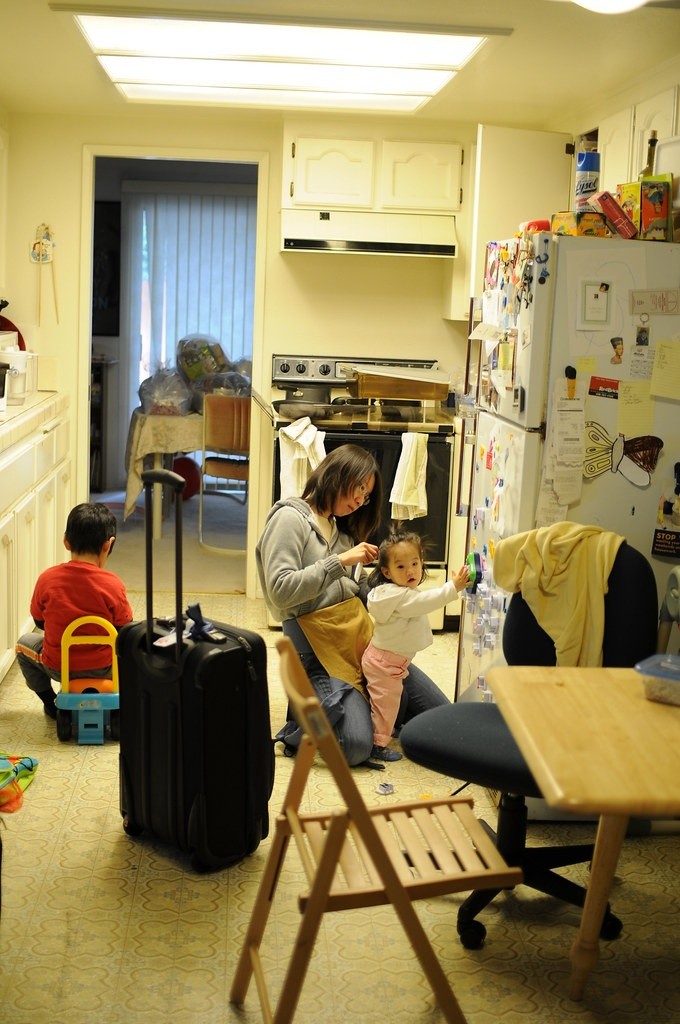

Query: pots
(277, 383), (332, 404)
(270, 399), (374, 420)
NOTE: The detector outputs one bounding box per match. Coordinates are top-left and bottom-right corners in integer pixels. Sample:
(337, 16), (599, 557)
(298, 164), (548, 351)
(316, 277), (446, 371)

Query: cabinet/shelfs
(1, 413), (72, 685)
(570, 85), (680, 195)
(273, 436), (455, 569)
(281, 117), (468, 211)
(92, 364), (119, 493)
(444, 122), (576, 322)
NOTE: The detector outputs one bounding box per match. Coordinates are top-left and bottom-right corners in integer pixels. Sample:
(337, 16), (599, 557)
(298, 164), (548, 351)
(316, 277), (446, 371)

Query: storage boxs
(553, 180), (669, 241)
(345, 370), (449, 400)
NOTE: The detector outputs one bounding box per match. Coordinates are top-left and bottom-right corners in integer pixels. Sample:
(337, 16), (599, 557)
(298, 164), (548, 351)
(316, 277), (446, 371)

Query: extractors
(279, 209), (459, 259)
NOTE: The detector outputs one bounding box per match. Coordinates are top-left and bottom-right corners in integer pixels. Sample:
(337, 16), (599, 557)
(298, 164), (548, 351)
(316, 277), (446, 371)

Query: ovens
(265, 431), (457, 629)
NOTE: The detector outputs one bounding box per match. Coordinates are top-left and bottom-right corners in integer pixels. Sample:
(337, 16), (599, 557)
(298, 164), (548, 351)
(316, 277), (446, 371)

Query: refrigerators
(458, 234), (680, 821)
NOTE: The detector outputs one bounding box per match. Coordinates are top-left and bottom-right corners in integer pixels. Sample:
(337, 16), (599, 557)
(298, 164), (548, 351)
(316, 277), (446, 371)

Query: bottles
(574, 151), (601, 211)
(636, 130), (659, 182)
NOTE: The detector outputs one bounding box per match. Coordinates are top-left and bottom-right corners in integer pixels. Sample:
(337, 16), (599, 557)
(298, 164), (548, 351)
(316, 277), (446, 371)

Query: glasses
(356, 483), (371, 506)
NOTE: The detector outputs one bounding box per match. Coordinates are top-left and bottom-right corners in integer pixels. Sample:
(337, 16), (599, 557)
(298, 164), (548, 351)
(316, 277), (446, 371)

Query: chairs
(199, 394), (251, 558)
(398, 519), (659, 952)
(228, 636), (524, 1024)
(57, 615), (120, 711)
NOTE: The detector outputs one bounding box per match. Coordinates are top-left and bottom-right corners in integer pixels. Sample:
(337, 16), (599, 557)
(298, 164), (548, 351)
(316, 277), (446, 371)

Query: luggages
(117, 468), (276, 874)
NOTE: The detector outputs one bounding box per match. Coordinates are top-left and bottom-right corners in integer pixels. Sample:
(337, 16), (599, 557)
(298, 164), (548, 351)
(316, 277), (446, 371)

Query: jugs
(0, 345), (39, 406)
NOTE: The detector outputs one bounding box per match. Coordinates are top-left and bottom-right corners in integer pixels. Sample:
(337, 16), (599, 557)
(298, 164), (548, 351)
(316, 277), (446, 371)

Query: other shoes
(44, 701), (57, 719)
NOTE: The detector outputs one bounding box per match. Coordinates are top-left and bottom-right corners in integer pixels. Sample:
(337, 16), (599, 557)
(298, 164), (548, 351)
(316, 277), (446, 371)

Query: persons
(636, 327), (648, 346)
(361, 532), (474, 761)
(256, 444), (452, 768)
(16, 503), (133, 718)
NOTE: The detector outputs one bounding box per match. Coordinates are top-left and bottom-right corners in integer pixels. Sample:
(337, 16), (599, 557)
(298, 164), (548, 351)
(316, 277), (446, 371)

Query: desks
(486, 665), (679, 1002)
(123, 406), (204, 538)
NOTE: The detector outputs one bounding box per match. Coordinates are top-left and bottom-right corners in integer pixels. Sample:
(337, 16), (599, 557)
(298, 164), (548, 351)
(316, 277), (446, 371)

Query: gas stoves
(269, 354), (455, 437)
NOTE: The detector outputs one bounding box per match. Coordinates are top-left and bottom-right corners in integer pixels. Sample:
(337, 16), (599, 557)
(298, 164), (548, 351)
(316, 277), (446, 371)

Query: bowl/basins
(634, 654), (680, 707)
